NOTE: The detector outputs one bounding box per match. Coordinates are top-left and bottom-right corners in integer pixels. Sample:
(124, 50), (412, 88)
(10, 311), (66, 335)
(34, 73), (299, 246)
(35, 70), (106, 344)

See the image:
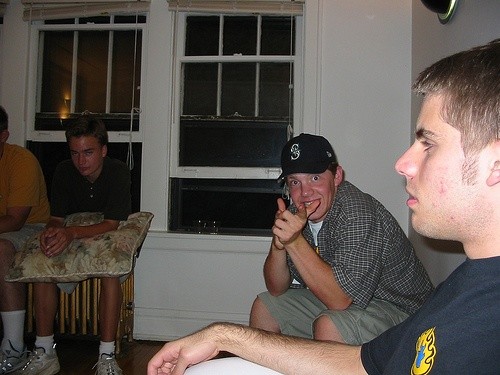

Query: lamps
(421, 0), (457, 24)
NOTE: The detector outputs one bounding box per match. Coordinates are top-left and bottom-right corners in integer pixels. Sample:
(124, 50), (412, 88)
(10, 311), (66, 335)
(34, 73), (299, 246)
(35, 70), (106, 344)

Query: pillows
(4, 212), (155, 284)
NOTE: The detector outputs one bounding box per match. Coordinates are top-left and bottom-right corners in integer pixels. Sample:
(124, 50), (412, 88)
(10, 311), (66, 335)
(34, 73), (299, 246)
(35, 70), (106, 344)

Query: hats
(277, 133), (336, 184)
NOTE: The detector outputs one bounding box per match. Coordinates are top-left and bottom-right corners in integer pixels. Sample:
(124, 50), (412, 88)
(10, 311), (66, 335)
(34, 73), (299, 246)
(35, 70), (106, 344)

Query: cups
(193, 220), (206, 234)
(208, 220), (221, 235)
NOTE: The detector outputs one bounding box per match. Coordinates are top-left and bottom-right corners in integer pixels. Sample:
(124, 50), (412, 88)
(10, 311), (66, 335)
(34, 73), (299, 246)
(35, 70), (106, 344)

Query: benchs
(25, 272), (135, 358)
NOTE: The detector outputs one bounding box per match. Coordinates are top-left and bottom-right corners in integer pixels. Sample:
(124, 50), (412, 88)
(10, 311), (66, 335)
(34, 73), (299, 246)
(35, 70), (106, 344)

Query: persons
(0, 105), (50, 375)
(149, 39), (500, 375)
(10, 117), (133, 375)
(249, 134), (434, 347)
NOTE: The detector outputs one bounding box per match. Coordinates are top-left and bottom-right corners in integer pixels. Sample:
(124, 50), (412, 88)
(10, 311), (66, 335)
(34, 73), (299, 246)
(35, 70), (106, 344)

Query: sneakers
(14, 342), (61, 375)
(91, 353), (122, 375)
(0, 338), (27, 374)
